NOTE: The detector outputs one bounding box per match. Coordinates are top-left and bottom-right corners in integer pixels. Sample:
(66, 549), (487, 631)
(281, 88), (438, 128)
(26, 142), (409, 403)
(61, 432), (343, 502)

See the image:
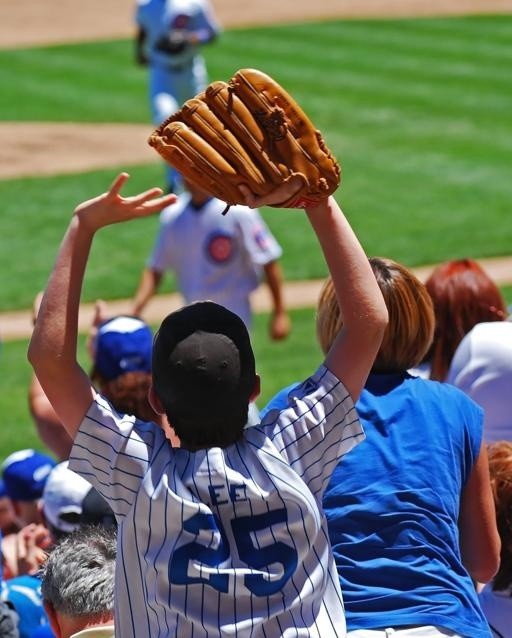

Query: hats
(42, 460), (115, 535)
(152, 300), (257, 418)
(0, 448), (56, 497)
(89, 317), (154, 381)
(0, 574), (55, 638)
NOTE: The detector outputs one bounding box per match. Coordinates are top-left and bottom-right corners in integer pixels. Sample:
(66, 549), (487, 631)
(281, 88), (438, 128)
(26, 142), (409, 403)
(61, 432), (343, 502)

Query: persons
(258, 257), (512, 638)
(134, 0), (220, 195)
(27, 176), (391, 636)
(127, 179), (293, 341)
(1, 313), (179, 638)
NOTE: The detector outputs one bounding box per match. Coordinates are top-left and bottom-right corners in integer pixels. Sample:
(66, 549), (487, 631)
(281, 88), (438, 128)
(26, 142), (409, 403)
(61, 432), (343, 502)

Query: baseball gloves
(148, 68), (341, 215)
